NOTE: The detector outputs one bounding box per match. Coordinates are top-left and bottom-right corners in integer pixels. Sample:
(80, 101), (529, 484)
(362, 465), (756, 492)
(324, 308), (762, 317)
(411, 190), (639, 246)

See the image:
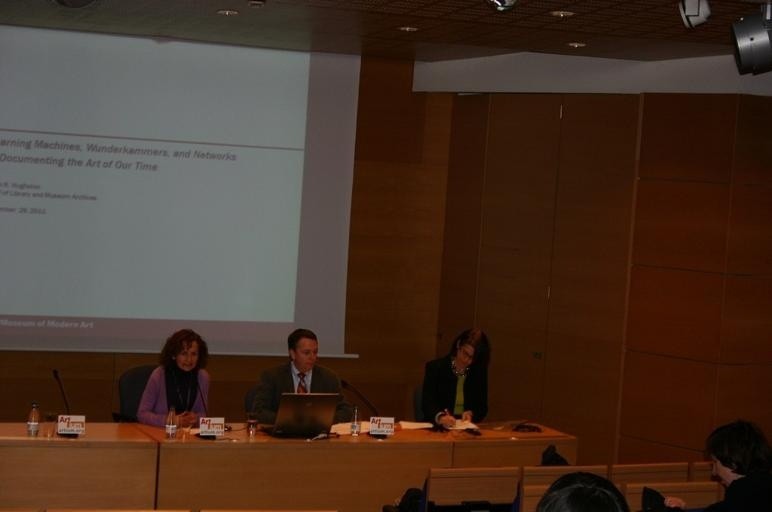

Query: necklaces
(453, 361), (471, 377)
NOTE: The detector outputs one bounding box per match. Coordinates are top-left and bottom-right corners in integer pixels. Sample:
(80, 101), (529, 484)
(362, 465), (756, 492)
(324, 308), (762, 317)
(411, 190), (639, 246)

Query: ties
(297, 373), (307, 394)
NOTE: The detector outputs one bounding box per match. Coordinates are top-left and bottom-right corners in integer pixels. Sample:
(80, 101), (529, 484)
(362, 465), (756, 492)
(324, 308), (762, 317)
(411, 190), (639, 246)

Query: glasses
(459, 348), (472, 360)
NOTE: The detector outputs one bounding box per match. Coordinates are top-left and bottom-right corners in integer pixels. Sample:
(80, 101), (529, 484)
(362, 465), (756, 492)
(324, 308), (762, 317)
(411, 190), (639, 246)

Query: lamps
(679, 0), (712, 31)
(728, 14), (772, 76)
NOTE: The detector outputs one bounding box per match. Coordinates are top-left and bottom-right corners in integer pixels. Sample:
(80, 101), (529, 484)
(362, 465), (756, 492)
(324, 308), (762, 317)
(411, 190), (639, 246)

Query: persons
(421, 327), (491, 426)
(249, 328), (352, 425)
(135, 328), (211, 428)
(664, 420), (772, 512)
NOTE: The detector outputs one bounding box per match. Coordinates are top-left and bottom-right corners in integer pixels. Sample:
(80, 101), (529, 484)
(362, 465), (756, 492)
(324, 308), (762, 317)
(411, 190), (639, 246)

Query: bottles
(26, 405), (40, 438)
(165, 407), (177, 441)
(350, 405), (362, 442)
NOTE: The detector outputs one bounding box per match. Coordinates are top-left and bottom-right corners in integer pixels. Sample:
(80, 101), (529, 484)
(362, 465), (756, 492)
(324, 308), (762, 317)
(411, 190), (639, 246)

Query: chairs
(112, 364), (163, 422)
(518, 483), (624, 512)
(422, 465), (520, 512)
(623, 481), (727, 512)
(690, 460), (721, 481)
(244, 381), (273, 426)
(612, 461), (689, 495)
(415, 383), (428, 422)
(518, 463), (611, 484)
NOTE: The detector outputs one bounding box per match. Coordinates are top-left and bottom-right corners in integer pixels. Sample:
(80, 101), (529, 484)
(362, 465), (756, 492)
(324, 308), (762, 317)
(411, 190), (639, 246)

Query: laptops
(261, 392), (339, 439)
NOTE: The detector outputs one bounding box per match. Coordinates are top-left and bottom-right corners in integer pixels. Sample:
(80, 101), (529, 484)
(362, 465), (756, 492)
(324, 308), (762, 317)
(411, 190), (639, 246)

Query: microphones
(52, 366), (71, 418)
(187, 369), (209, 419)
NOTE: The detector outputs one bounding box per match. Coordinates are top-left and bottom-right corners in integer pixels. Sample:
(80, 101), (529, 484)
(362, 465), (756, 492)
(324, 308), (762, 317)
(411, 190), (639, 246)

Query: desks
(448, 417), (577, 504)
(136, 421), (455, 511)
(0, 422), (159, 512)
